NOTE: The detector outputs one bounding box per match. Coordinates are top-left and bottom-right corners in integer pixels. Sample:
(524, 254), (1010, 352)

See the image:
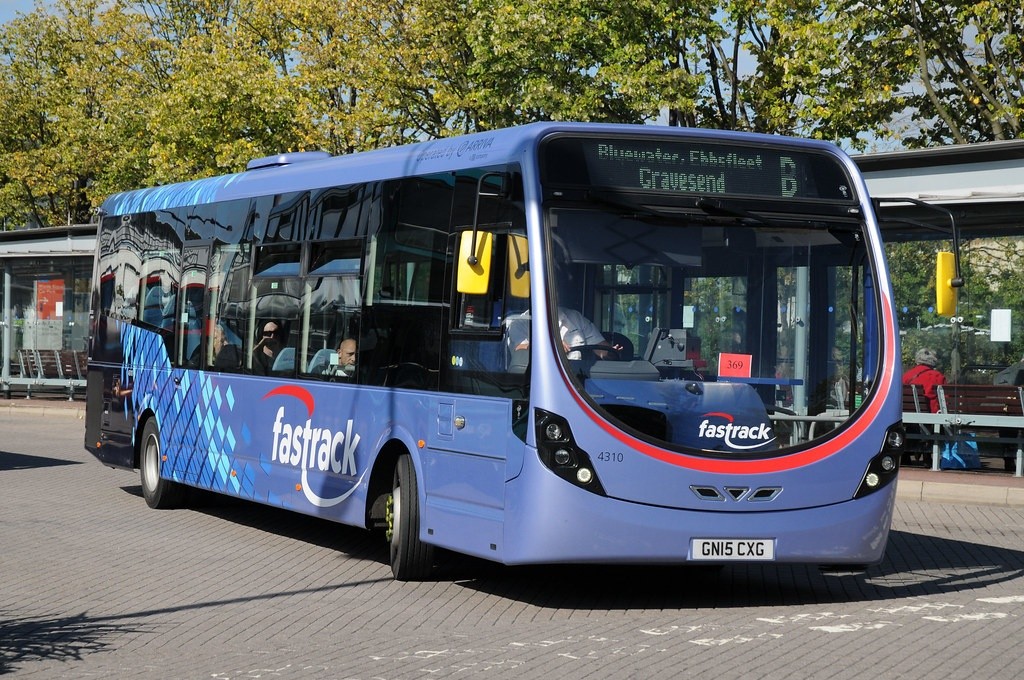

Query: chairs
(143, 286), (173, 322)
(271, 348), (295, 372)
(308, 348), (338, 375)
(161, 294), (195, 333)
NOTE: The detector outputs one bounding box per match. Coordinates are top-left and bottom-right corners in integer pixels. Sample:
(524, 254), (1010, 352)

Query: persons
(209, 324), (240, 372)
(252, 318), (295, 377)
(993, 355), (1024, 471)
(505, 273), (624, 373)
(321, 338), (356, 378)
(899, 348), (950, 468)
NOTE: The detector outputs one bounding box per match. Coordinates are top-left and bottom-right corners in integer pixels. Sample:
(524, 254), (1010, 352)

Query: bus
(83, 121), (961, 581)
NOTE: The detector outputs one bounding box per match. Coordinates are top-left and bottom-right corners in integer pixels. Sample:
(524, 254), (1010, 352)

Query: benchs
(900, 384), (1023, 478)
(16, 349), (88, 401)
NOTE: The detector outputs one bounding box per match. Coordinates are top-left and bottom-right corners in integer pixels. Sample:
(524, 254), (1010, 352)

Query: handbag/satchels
(939, 429), (983, 470)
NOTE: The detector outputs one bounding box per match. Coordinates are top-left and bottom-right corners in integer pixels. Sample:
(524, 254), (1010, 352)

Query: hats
(915, 349), (938, 368)
